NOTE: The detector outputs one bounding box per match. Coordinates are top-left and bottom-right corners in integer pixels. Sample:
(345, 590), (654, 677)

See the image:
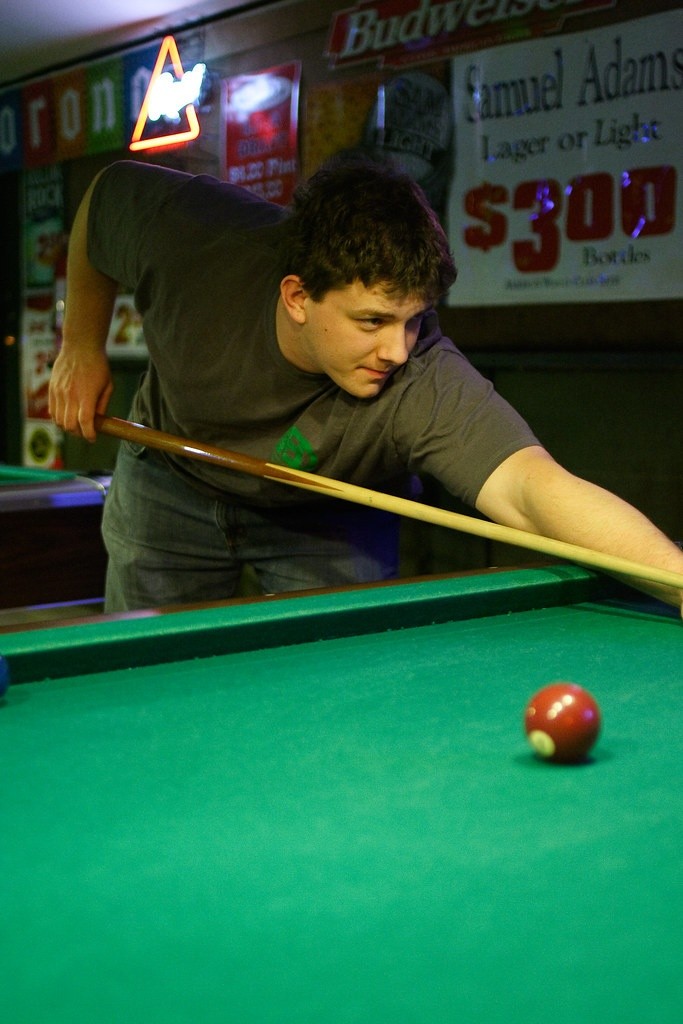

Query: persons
(48, 149), (682, 614)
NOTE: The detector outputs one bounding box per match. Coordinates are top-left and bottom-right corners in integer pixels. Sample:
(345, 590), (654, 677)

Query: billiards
(523, 680), (601, 762)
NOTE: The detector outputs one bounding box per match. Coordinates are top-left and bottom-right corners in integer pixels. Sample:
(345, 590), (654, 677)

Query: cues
(88, 412), (683, 590)
